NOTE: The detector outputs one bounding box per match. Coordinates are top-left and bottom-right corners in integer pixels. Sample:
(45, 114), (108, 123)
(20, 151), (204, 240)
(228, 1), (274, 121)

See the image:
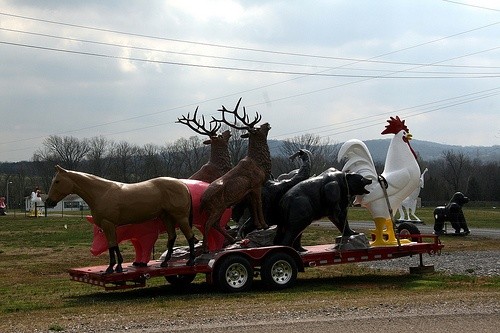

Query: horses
(46, 164), (196, 273)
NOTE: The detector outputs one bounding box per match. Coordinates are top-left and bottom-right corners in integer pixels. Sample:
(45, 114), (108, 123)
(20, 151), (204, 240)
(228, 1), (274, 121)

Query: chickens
(337, 116), (420, 246)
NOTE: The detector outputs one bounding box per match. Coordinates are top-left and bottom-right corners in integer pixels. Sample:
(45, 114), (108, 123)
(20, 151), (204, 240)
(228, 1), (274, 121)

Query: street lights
(7, 176), (12, 208)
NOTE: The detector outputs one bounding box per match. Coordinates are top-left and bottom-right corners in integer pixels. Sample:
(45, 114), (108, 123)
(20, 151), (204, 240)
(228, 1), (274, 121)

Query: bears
(273, 173), (372, 252)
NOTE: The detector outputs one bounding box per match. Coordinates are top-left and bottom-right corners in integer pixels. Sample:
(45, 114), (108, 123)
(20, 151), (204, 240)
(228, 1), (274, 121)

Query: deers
(199, 97), (272, 255)
(175, 105), (233, 185)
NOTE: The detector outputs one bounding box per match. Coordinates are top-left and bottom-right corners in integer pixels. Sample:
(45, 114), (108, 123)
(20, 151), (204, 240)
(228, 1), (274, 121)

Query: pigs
(85, 178), (232, 268)
